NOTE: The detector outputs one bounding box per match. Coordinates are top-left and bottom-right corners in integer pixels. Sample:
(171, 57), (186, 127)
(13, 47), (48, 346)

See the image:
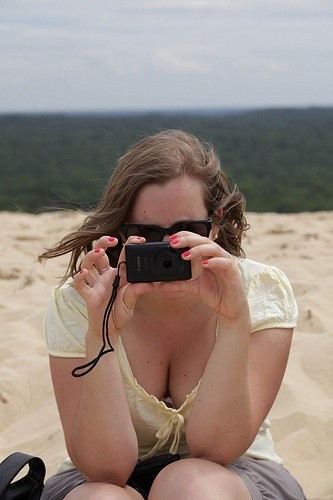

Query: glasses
(118, 218), (212, 243)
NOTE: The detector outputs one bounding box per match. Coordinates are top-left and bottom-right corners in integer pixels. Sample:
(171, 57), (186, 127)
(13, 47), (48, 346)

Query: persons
(35, 127), (308, 500)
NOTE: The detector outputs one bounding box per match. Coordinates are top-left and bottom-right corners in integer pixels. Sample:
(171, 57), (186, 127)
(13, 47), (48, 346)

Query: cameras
(124, 241), (191, 283)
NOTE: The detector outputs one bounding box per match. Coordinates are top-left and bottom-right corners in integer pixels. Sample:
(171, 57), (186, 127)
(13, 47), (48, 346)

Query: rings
(100, 264), (111, 273)
